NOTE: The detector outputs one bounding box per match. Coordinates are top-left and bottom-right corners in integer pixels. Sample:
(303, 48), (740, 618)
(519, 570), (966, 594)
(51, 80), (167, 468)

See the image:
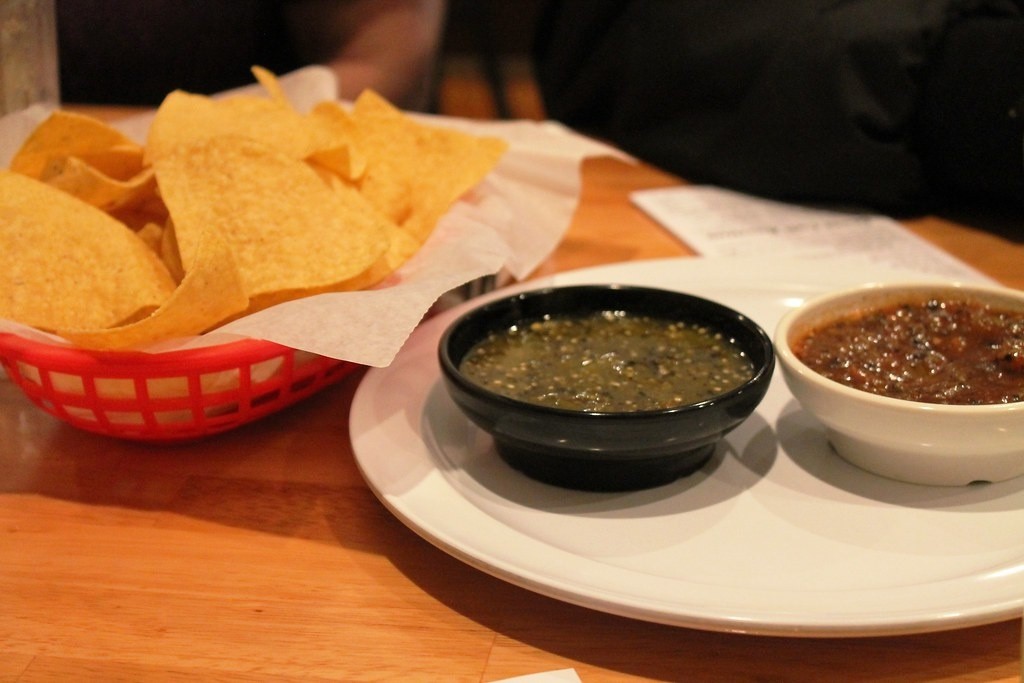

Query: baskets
(1, 331), (388, 440)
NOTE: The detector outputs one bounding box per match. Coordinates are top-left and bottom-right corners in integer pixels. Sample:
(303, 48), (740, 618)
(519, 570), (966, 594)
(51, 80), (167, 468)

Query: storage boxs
(0, 333), (358, 441)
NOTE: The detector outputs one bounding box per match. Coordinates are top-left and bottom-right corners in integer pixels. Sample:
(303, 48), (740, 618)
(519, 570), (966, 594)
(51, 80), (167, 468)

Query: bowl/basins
(437, 285), (775, 493)
(774, 279), (1022, 490)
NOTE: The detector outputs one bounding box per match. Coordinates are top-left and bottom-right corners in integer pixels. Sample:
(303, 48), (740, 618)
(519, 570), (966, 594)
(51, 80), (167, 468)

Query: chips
(0, 63), (509, 350)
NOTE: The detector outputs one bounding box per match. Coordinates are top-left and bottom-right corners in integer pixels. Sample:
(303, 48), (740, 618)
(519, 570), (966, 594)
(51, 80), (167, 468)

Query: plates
(349, 250), (1022, 632)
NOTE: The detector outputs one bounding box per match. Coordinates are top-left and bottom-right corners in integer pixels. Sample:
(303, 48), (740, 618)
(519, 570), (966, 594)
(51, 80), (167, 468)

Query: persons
(55, 0), (445, 141)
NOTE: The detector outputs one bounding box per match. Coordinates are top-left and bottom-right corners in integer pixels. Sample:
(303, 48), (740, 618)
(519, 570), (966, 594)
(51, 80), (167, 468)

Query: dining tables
(1, 106), (1024, 683)
